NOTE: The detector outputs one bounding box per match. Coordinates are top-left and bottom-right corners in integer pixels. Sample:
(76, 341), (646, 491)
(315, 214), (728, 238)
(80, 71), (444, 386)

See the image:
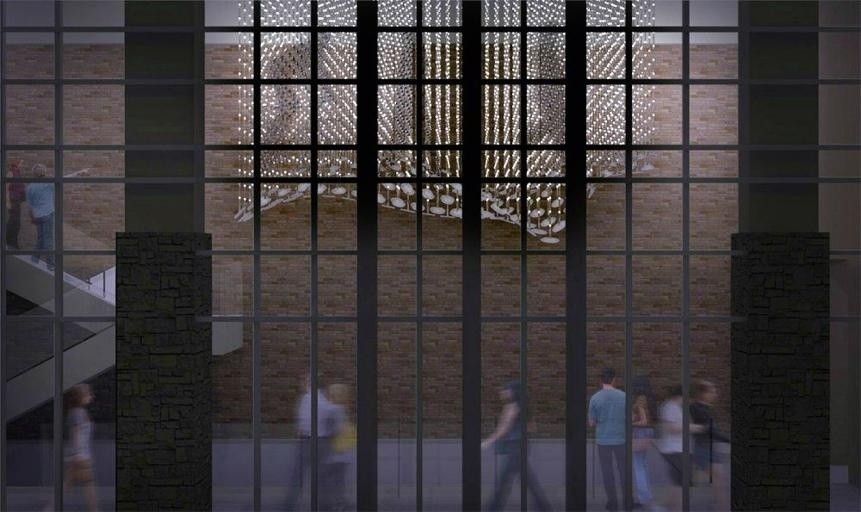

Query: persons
(479, 378), (552, 511)
(624, 370), (660, 511)
(318, 380), (356, 510)
(656, 381), (695, 511)
(2, 154), (28, 252)
(63, 379), (101, 512)
(587, 364), (627, 510)
(25, 161), (90, 271)
(282, 369), (332, 510)
(685, 378), (729, 512)
